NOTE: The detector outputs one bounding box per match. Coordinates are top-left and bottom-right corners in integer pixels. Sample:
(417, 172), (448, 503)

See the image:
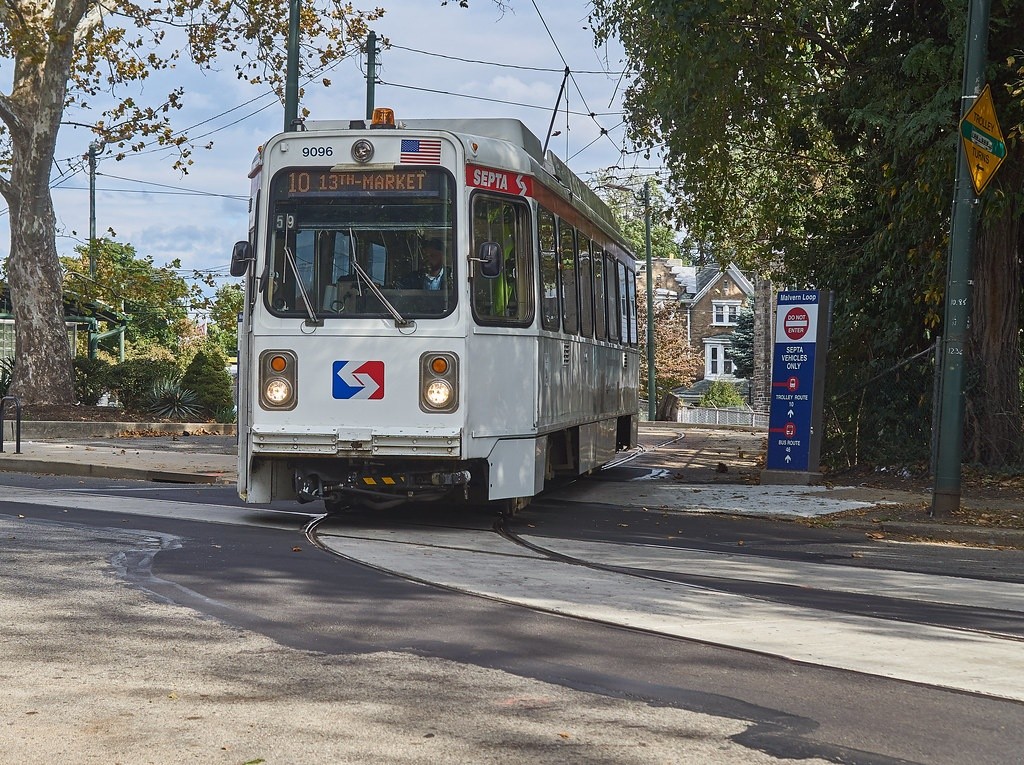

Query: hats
(421, 239), (448, 252)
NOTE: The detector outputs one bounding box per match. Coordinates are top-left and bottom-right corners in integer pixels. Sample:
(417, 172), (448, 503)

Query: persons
(374, 239), (453, 291)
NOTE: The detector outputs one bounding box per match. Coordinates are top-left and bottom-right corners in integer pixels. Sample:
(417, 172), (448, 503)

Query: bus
(231, 116), (642, 514)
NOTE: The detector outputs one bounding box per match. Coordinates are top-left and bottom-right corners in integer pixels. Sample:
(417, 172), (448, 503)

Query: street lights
(606, 183), (657, 423)
(88, 140), (107, 360)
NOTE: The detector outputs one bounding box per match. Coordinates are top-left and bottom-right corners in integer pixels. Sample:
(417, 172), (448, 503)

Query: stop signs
(785, 307), (809, 341)
